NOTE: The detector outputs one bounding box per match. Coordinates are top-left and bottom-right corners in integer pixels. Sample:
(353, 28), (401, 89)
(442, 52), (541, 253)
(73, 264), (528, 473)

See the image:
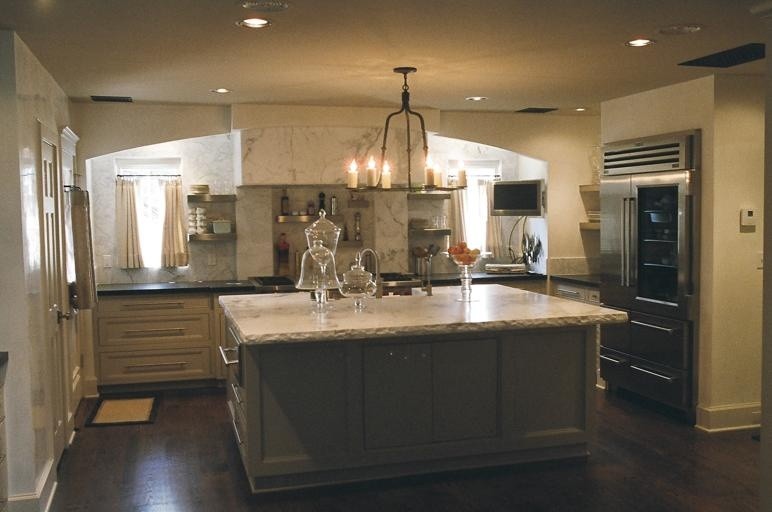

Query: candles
(345, 154), (467, 194)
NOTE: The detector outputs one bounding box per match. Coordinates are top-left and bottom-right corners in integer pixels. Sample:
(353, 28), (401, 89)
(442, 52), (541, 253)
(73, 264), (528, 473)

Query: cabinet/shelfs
(555, 283), (606, 390)
(96, 292), (213, 392)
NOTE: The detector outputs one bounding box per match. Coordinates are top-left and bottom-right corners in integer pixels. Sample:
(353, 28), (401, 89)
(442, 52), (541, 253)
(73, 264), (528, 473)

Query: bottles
(281, 186), (290, 216)
(276, 233), (290, 275)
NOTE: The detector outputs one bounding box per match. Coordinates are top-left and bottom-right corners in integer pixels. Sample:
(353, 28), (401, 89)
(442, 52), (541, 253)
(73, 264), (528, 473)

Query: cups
(430, 216), (447, 229)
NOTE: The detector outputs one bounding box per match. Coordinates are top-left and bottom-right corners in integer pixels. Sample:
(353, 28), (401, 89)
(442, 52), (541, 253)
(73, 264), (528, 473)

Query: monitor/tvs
(488, 179), (542, 216)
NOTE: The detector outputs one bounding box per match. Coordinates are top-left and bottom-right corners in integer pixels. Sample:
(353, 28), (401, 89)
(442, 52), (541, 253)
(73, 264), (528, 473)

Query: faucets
(356, 247), (382, 285)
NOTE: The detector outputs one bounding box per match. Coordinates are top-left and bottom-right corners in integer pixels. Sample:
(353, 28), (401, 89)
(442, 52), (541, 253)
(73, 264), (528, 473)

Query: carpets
(84, 398), (160, 425)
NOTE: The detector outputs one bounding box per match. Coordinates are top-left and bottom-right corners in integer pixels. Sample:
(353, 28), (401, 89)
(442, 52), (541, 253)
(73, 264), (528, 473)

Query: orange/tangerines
(447, 241), (481, 265)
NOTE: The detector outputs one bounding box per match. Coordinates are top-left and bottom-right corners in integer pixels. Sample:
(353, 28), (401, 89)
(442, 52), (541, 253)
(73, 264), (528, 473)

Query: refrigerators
(597, 169), (699, 410)
